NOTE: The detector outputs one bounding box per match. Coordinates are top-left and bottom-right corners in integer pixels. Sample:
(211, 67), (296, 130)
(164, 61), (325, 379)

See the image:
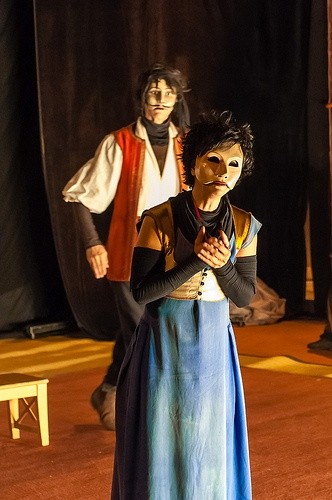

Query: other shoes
(91, 382), (117, 430)
(307, 338), (332, 350)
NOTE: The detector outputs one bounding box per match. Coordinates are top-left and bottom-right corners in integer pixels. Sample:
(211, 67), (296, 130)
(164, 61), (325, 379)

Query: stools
(0, 372), (51, 450)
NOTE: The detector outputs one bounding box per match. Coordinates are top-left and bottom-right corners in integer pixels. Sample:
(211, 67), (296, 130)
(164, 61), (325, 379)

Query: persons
(309, 278), (332, 351)
(110, 109), (262, 500)
(62, 64), (192, 430)
